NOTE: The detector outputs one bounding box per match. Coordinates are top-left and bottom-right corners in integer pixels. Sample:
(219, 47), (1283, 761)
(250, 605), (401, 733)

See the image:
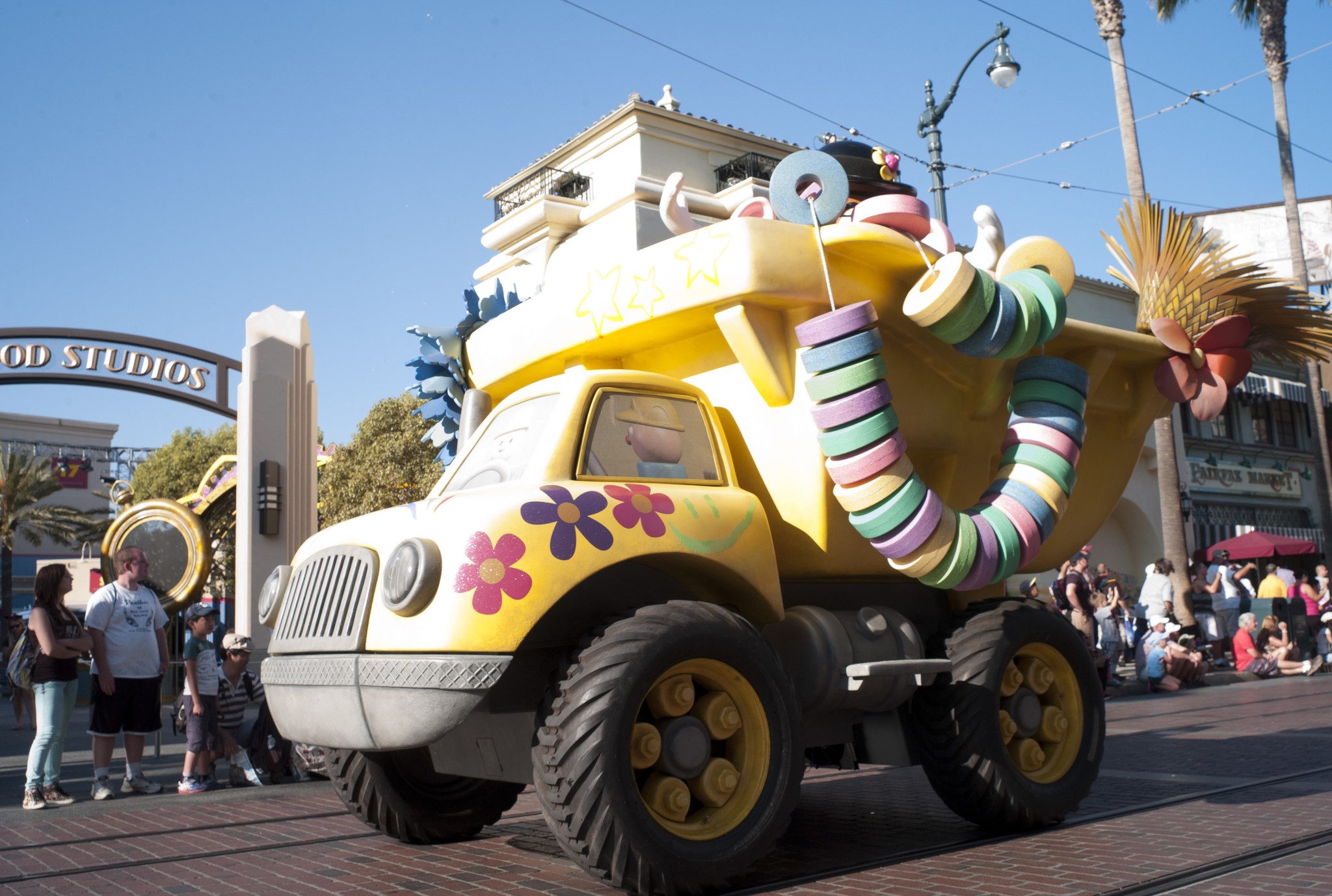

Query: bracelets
(1109, 594), (1114, 596)
(1281, 630), (1288, 633)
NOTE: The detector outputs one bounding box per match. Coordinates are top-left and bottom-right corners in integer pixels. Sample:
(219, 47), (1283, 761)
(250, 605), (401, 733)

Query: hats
(1320, 611), (1332, 622)
(1149, 615), (1195, 646)
(1020, 577), (1037, 593)
(1213, 548), (1229, 560)
(184, 602), (220, 621)
(220, 634), (256, 652)
(1070, 550), (1091, 563)
(1081, 545), (1092, 552)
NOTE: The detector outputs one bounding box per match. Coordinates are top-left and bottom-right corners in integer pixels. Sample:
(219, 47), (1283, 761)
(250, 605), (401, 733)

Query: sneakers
(120, 772), (161, 793)
(90, 775), (114, 799)
(178, 778), (208, 794)
(43, 781), (77, 803)
(199, 775), (226, 789)
(22, 787), (47, 809)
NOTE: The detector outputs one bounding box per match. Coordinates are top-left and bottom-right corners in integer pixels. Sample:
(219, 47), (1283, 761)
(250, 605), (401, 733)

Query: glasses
(227, 637), (251, 649)
(10, 623), (21, 629)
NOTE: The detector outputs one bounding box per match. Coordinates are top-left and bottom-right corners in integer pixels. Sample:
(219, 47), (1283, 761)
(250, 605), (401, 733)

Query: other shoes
(1306, 655), (1323, 675)
(1102, 660), (1232, 696)
(13, 725), (22, 730)
(31, 724), (36, 729)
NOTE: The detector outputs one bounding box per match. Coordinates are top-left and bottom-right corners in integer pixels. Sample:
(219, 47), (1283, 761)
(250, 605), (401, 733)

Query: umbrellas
(1193, 530), (1318, 587)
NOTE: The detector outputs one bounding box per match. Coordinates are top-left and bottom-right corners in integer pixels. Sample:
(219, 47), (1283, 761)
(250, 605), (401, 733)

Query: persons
(0, 564), (94, 810)
(82, 546), (170, 801)
(1020, 545), (1332, 700)
(177, 602), (226, 793)
(206, 633), (292, 789)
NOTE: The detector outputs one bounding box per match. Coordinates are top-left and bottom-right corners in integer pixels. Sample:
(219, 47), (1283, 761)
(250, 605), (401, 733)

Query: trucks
(261, 206), (1257, 896)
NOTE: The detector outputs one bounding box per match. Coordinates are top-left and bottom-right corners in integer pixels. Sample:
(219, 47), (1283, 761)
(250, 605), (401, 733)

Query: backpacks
(1049, 572), (1081, 610)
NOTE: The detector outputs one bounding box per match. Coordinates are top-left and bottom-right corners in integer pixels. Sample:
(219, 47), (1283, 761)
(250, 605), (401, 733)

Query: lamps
(57, 457), (69, 471)
(98, 476), (116, 484)
(1186, 441), (1217, 467)
(1285, 455), (1311, 481)
(1179, 480), (1193, 522)
(1253, 449), (1283, 472)
(814, 131), (836, 150)
(1220, 444), (1252, 469)
(78, 459), (93, 472)
(257, 459), (282, 535)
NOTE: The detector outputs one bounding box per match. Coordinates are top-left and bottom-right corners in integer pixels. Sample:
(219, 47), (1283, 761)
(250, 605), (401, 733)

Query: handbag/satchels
(170, 689), (187, 736)
(6, 629), (41, 689)
(1124, 617), (1136, 648)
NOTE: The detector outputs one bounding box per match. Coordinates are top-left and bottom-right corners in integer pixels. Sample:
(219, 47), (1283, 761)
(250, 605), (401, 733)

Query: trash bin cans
(1240, 596), (1310, 647)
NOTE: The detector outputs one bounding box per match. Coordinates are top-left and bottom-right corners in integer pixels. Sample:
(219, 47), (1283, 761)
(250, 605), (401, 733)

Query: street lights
(915, 20), (1021, 227)
